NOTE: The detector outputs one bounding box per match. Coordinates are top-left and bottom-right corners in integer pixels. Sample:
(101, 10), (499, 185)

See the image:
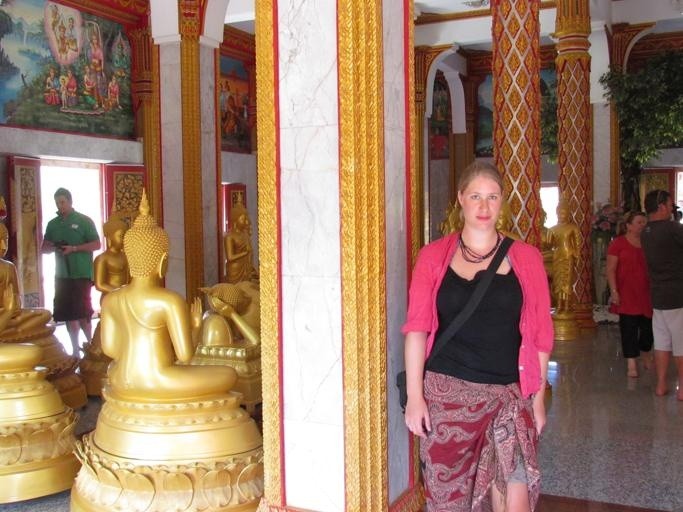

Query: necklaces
(458, 231), (501, 265)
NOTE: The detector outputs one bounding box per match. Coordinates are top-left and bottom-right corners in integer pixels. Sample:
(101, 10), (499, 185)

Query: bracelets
(71, 245), (78, 254)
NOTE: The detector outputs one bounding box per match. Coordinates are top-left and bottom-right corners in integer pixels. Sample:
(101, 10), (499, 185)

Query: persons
(219, 78), (250, 140)
(42, 5), (130, 112)
(40, 187), (101, 363)
(220, 191), (255, 284)
(90, 217), (126, 347)
(202, 279), (259, 346)
(401, 161), (552, 512)
(606, 211), (652, 378)
(0, 220), (51, 340)
(99, 186), (238, 401)
(547, 199), (581, 314)
(640, 189), (683, 402)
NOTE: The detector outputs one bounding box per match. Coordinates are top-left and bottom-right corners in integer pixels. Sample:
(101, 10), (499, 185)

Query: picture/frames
(429, 78), (449, 159)
(0, 0), (138, 142)
(639, 168), (675, 217)
(220, 50), (252, 154)
(474, 69), (557, 157)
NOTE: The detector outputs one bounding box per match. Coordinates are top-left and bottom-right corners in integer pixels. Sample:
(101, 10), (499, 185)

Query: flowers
(592, 205), (627, 244)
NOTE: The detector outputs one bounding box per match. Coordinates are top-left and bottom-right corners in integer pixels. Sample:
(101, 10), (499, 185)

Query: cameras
(54, 240), (68, 256)
